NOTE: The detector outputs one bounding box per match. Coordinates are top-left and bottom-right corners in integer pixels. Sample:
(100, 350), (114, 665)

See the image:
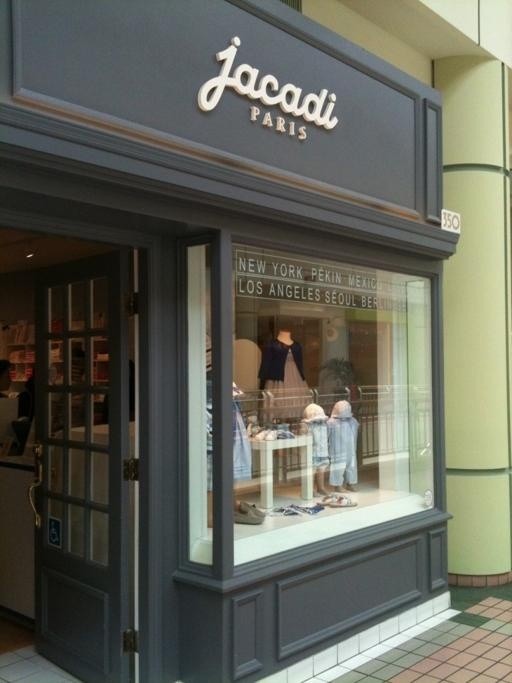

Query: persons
(299, 400), (360, 497)
(0, 359), (12, 398)
(259, 330), (311, 419)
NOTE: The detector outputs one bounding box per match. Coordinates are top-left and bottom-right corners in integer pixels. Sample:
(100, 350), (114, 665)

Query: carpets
(1, 620), (38, 656)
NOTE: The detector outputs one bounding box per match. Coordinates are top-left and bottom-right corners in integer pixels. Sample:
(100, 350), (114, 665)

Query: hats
(330, 400), (353, 418)
(300, 403), (329, 423)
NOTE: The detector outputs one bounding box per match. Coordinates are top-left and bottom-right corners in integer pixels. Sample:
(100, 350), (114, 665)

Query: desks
(246, 433), (315, 508)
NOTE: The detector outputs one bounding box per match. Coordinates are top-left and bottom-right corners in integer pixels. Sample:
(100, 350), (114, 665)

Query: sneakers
(255, 428), (278, 440)
(234, 502), (265, 525)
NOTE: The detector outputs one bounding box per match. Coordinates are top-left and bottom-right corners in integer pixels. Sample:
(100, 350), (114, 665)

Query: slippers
(316, 492), (357, 507)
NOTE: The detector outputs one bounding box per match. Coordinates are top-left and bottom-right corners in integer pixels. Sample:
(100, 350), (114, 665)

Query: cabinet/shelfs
(7, 336), (109, 382)
